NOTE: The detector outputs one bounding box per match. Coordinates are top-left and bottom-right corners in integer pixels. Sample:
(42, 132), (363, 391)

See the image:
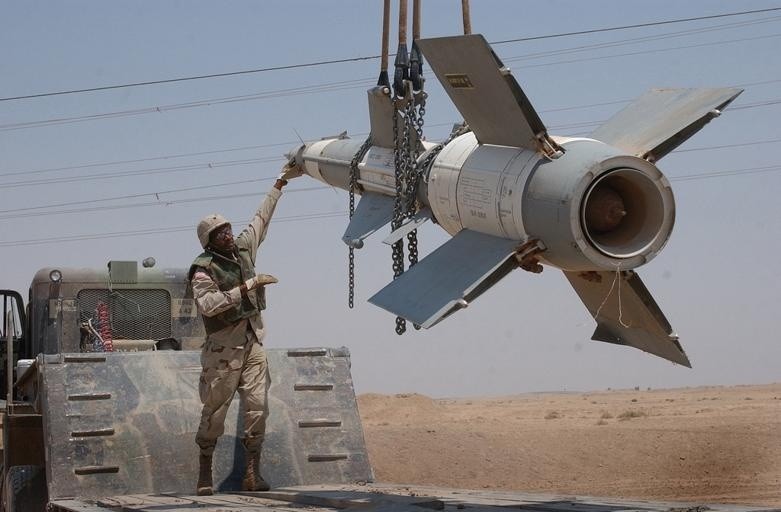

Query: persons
(188, 155), (297, 496)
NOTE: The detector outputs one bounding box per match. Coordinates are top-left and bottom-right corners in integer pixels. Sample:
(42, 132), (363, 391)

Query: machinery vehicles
(0, 257), (780, 511)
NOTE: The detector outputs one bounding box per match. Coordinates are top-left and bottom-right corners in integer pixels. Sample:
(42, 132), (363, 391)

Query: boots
(242, 452), (269, 491)
(197, 454), (213, 495)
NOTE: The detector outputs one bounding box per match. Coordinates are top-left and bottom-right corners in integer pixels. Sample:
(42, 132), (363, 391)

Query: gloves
(245, 273), (278, 291)
(277, 157), (305, 182)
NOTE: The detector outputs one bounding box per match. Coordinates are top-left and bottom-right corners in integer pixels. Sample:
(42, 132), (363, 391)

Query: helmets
(197, 213), (232, 249)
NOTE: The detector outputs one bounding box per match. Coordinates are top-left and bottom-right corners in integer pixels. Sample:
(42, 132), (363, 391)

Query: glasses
(213, 228), (231, 240)
